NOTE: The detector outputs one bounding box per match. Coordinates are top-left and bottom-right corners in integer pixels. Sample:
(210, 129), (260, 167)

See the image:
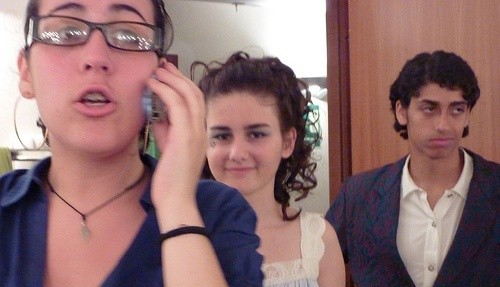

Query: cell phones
(143, 89), (166, 122)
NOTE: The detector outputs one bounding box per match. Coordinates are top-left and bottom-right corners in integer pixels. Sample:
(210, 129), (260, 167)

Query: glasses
(32, 15), (163, 52)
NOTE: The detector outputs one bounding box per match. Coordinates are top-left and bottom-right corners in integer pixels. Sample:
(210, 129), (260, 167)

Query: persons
(325, 50), (500, 287)
(189, 50), (346, 287)
(1, 0), (265, 287)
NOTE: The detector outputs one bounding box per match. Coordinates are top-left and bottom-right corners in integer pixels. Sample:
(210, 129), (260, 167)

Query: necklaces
(45, 167), (147, 241)
